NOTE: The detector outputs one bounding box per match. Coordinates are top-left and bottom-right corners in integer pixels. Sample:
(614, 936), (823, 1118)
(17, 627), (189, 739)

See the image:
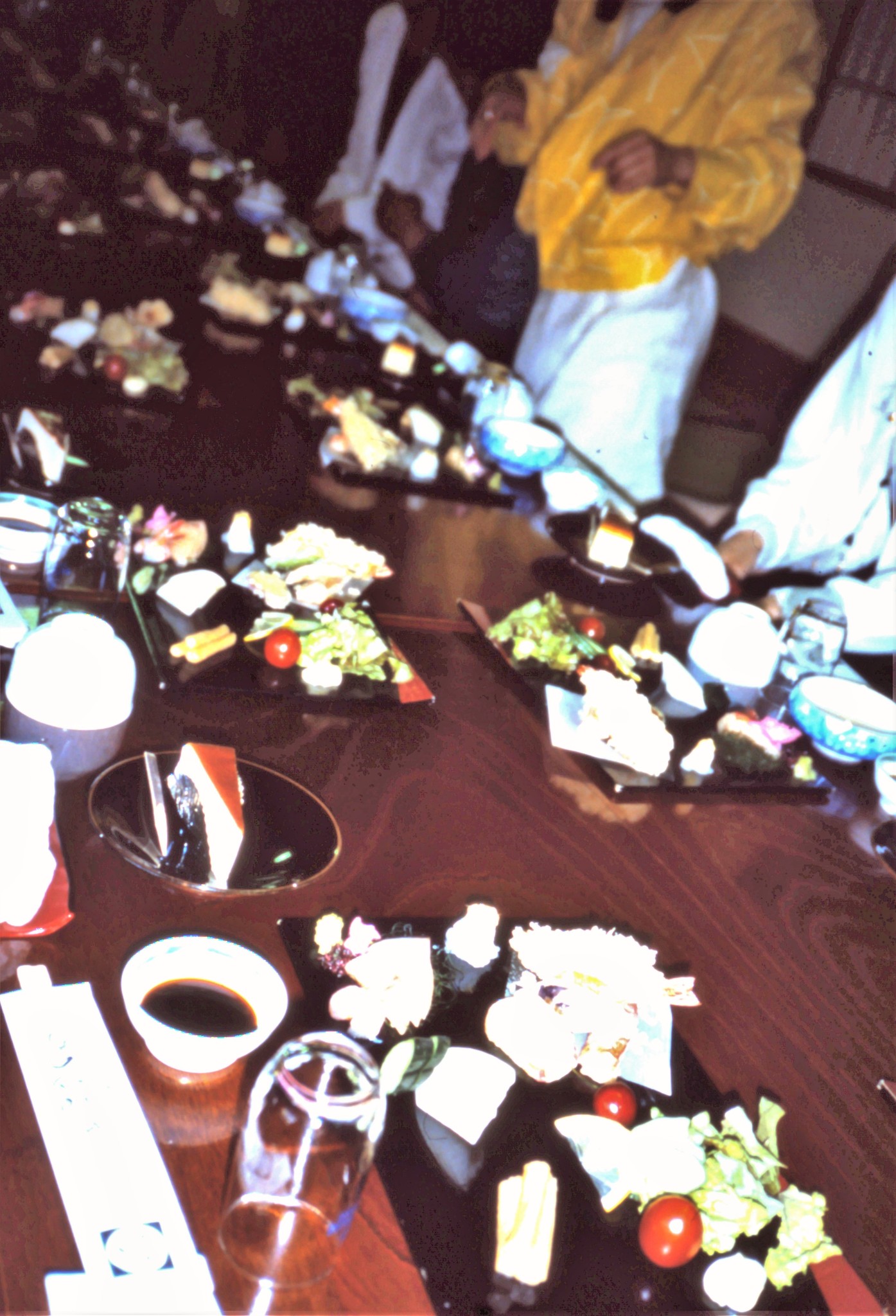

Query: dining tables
(1, 1), (894, 1315)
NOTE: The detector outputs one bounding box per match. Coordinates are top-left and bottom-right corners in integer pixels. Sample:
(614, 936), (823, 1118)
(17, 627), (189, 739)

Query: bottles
(765, 597), (848, 709)
(44, 498), (132, 599)
(218, 1031), (387, 1292)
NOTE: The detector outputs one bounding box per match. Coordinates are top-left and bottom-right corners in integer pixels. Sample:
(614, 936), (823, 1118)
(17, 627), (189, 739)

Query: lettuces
(647, 1094), (843, 1289)
(485, 590), (600, 674)
(283, 601), (414, 683)
(117, 347), (191, 392)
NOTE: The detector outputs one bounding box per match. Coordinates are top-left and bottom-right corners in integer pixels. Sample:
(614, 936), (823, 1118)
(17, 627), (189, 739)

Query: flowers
(134, 507), (195, 568)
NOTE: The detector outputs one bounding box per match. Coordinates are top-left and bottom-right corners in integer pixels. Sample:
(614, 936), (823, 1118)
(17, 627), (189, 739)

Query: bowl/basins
(543, 469), (599, 512)
(88, 751), (341, 900)
(341, 286), (419, 346)
(0, 501), (55, 564)
(355, 339), (438, 395)
(482, 420), (563, 477)
(790, 675), (896, 763)
(688, 602), (780, 688)
(6, 612), (135, 731)
(545, 512), (681, 583)
(240, 198), (282, 226)
(121, 936), (288, 1073)
(875, 753), (896, 815)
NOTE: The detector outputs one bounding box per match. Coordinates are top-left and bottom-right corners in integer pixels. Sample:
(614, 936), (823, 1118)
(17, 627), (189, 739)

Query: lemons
(243, 612), (293, 642)
(554, 1113), (642, 1212)
(607, 643), (642, 680)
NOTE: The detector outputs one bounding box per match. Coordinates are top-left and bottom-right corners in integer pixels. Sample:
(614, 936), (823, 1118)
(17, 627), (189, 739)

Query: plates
(457, 597), (838, 803)
(280, 915), (832, 1316)
(123, 493), (437, 718)
(280, 361), (516, 507)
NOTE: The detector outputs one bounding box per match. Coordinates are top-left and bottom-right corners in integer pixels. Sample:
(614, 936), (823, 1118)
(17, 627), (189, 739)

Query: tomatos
(592, 1082), (636, 1127)
(639, 1195), (702, 1268)
(579, 616), (606, 642)
(104, 355), (128, 382)
(263, 628), (300, 668)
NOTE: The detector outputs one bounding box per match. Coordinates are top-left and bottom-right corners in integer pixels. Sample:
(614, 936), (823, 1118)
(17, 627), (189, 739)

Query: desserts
(587, 500), (636, 570)
(12, 406), (72, 486)
(165, 742), (245, 887)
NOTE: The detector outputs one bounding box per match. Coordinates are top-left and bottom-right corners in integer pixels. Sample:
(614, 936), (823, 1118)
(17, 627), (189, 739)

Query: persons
(312, 0), (827, 505)
(719, 274), (896, 702)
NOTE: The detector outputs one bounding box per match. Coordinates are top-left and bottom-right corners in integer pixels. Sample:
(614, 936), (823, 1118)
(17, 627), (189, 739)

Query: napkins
(545, 684), (628, 766)
(615, 997), (674, 1099)
